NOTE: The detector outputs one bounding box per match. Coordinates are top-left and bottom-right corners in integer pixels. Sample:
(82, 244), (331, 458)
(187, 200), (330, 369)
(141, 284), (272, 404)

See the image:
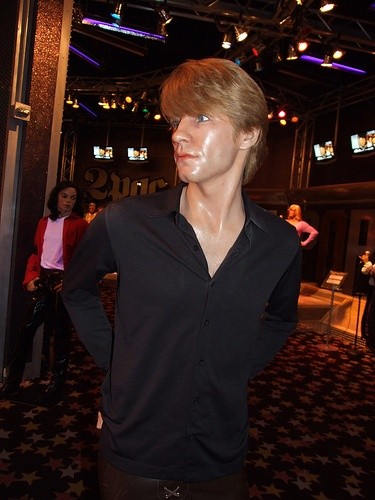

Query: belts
(98, 466), (249, 499)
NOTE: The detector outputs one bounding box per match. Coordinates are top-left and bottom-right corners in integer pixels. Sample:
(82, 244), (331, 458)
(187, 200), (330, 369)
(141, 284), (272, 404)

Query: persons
(85, 202), (102, 224)
(279, 204), (319, 247)
(358, 251), (375, 354)
(61, 58), (302, 500)
(0, 180), (88, 397)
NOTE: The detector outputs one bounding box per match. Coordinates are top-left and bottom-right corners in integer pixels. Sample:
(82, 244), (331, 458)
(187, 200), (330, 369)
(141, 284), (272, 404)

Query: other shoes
(2, 377), (20, 394)
(45, 371), (65, 393)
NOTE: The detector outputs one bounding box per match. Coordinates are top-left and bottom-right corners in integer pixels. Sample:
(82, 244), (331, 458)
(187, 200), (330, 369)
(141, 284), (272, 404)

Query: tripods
(314, 270), (349, 349)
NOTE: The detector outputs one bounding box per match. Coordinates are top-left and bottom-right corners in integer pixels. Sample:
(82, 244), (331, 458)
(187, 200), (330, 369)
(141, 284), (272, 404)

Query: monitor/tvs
(94, 146), (114, 161)
(314, 140), (336, 164)
(351, 130), (375, 158)
(128, 148), (149, 163)
